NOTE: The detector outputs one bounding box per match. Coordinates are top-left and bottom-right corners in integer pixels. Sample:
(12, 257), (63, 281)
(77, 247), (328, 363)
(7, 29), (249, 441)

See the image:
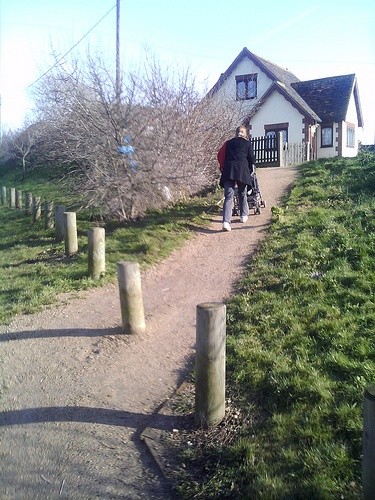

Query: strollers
(232, 164), (266, 214)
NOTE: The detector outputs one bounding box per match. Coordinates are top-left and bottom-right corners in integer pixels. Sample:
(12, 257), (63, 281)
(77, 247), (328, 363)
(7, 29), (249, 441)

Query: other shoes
(239, 216), (248, 222)
(222, 222), (231, 231)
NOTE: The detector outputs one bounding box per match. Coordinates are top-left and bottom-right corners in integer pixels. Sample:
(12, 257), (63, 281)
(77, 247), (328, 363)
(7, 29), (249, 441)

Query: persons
(217, 126), (257, 232)
